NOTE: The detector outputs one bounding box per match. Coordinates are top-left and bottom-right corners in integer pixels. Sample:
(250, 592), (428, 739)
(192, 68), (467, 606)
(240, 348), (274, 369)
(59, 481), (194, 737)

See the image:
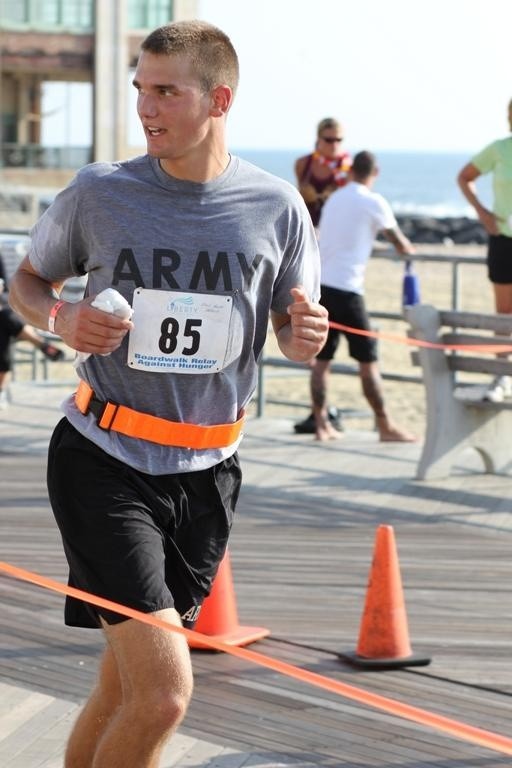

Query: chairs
(0, 232), (84, 384)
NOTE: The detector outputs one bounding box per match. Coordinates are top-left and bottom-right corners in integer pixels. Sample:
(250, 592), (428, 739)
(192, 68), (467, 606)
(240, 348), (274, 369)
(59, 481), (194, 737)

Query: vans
(0, 189), (83, 313)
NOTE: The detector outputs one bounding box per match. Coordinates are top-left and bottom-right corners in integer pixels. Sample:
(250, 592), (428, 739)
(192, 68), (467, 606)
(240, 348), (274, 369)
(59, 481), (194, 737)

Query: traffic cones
(180, 533), (271, 652)
(340, 521), (434, 673)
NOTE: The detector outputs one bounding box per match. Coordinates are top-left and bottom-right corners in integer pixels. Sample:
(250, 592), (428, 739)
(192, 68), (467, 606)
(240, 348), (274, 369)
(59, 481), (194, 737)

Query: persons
(1, 257), (63, 392)
(311, 152), (418, 442)
(295, 117), (351, 225)
(457, 98), (512, 403)
(9, 20), (329, 768)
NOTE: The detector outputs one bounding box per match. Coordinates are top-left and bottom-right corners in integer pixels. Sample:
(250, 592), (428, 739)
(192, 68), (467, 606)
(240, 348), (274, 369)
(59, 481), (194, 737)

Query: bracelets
(48, 299), (67, 333)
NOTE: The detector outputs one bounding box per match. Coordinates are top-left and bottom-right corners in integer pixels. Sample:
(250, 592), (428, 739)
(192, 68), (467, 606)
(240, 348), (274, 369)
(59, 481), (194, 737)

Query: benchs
(402, 304), (512, 481)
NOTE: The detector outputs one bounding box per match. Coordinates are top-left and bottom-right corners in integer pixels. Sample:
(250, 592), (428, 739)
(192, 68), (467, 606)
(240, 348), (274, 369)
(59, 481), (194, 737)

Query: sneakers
(484, 374), (511, 402)
(39, 343), (63, 362)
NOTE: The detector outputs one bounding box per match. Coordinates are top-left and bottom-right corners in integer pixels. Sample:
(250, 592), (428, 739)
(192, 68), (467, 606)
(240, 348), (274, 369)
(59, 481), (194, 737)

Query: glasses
(320, 136), (342, 143)
